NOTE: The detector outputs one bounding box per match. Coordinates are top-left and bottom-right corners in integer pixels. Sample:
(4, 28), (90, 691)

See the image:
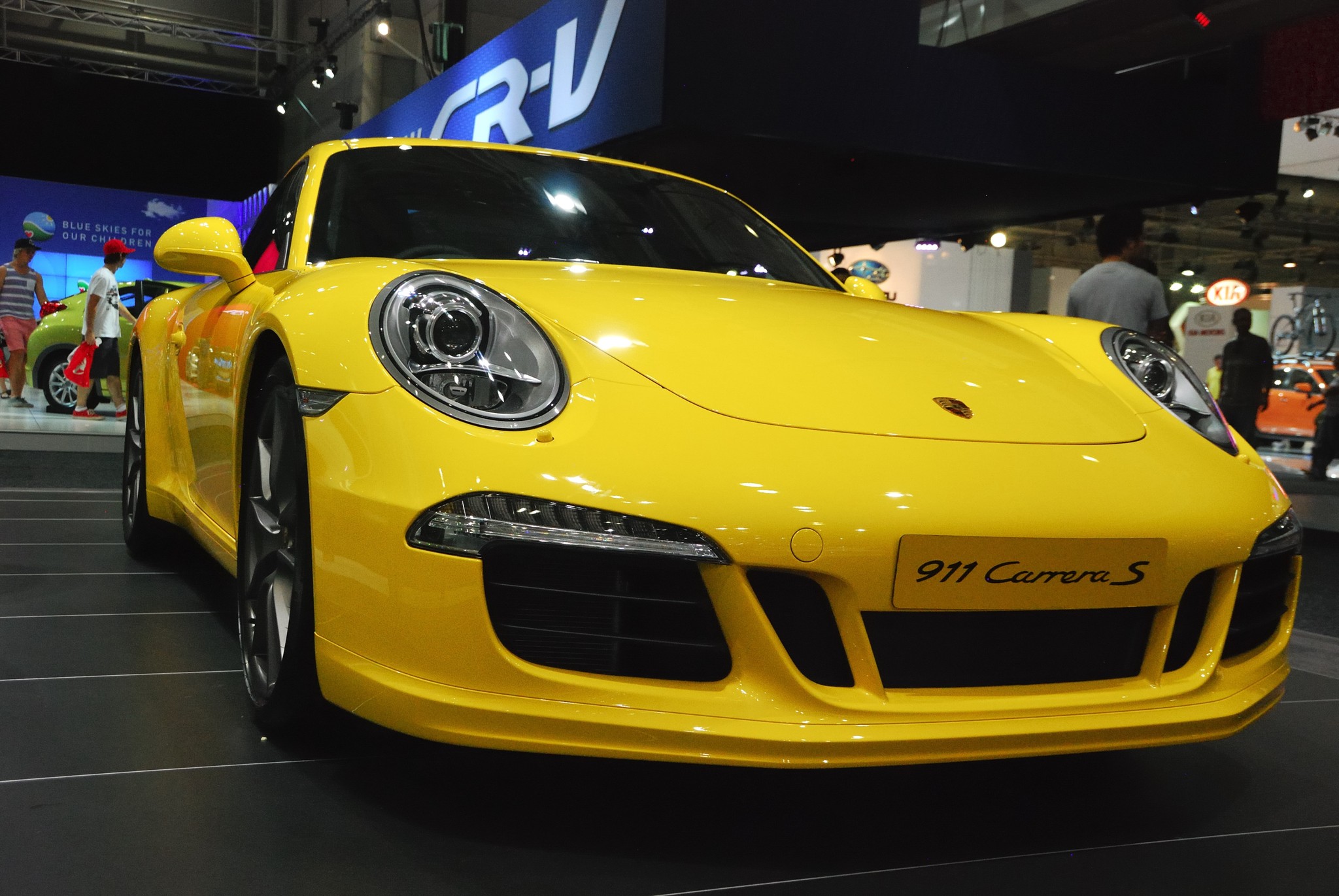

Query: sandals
(0, 389), (11, 398)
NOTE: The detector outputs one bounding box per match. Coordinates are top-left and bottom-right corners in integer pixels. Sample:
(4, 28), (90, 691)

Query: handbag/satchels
(65, 337), (102, 387)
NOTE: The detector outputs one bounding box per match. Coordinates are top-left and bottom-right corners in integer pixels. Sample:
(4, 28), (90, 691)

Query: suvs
(1254, 353), (1336, 449)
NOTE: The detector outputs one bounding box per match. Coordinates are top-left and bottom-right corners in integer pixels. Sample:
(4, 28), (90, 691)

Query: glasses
(21, 249), (36, 255)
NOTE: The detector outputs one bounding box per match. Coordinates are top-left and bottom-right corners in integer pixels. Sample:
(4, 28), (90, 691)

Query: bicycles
(1269, 291), (1336, 357)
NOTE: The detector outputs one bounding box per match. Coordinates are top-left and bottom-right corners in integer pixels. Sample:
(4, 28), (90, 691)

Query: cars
(24, 278), (203, 415)
(124, 140), (1302, 774)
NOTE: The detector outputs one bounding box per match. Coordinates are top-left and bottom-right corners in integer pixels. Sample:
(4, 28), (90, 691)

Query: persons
(73, 239), (136, 420)
(1307, 353), (1339, 476)
(0, 239), (48, 407)
(1206, 307), (1273, 442)
(1067, 210), (1173, 346)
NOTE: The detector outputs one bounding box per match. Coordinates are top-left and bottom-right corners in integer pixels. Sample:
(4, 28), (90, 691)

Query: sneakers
(115, 408), (128, 421)
(8, 395), (23, 406)
(21, 398), (34, 408)
(73, 408), (105, 420)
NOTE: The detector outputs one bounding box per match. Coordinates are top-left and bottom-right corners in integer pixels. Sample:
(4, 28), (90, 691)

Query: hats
(103, 239), (136, 254)
(15, 238), (41, 250)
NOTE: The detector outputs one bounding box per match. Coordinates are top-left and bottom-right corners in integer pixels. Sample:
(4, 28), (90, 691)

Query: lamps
(830, 249), (844, 265)
(312, 55), (338, 89)
